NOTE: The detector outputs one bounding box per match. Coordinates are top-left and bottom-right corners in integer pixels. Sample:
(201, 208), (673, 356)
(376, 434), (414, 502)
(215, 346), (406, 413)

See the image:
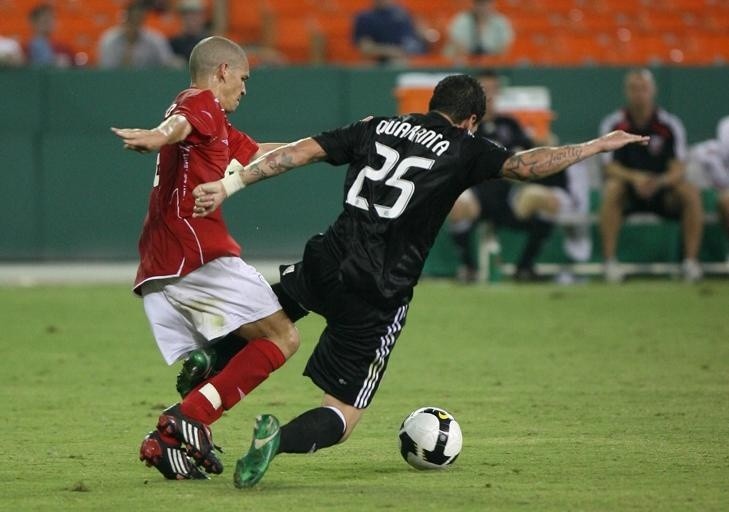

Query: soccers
(399, 407), (463, 470)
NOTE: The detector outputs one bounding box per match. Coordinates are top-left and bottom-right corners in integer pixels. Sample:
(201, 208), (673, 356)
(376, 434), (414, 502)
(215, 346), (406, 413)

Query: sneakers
(140, 403), (224, 481)
(232, 414), (281, 489)
(176, 347), (218, 399)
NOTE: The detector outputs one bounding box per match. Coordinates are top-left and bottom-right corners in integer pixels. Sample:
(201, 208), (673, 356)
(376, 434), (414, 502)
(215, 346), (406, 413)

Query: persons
(1, 0), (215, 69)
(111, 36), (304, 479)
(444, 68), (729, 287)
(175, 74), (650, 487)
(351, 1), (514, 66)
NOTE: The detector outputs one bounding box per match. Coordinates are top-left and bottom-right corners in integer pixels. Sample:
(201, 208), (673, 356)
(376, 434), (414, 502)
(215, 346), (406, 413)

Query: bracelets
(221, 171), (246, 198)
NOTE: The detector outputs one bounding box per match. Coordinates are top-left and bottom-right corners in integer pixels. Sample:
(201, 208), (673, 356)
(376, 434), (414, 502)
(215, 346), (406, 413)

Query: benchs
(469, 213), (728, 284)
